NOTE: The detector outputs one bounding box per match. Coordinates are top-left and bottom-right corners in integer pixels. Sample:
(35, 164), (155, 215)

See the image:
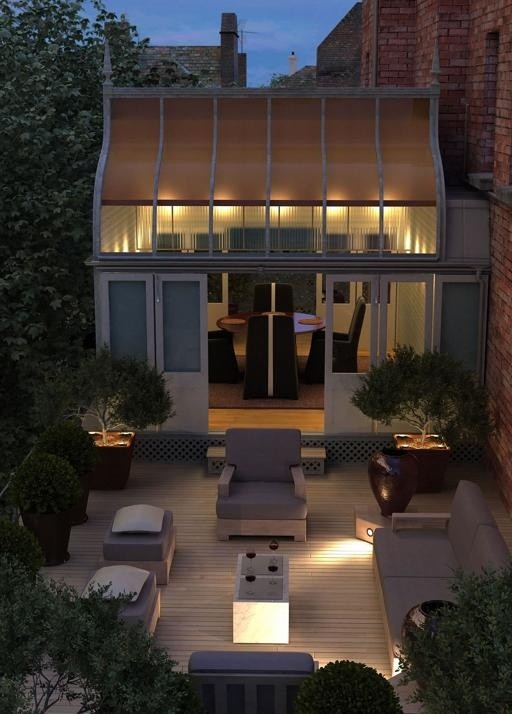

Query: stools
(80, 565), (161, 638)
(103, 507), (177, 586)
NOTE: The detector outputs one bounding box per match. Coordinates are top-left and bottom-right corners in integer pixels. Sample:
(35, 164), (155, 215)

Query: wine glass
(269, 539), (280, 565)
(266, 560), (278, 585)
(245, 570), (257, 594)
(245, 549), (256, 573)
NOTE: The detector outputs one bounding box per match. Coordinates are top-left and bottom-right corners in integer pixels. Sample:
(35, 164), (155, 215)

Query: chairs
(305, 296), (366, 382)
(254, 283), (292, 311)
(242, 314), (300, 400)
(208, 331), (240, 384)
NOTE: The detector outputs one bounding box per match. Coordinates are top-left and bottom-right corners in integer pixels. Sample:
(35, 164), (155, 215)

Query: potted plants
(51, 429), (95, 525)
(65, 343), (173, 490)
(12, 456), (71, 566)
(348, 344), (495, 517)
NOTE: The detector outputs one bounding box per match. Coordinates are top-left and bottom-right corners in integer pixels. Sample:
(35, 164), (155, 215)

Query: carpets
(209, 355), (368, 410)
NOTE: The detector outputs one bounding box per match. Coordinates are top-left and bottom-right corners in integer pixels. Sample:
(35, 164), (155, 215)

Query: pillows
(80, 566), (150, 601)
(112, 504), (164, 534)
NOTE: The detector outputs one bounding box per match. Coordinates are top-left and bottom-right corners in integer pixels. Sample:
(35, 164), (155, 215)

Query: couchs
(372, 481), (512, 679)
(216, 428), (308, 542)
(188, 650), (319, 713)
